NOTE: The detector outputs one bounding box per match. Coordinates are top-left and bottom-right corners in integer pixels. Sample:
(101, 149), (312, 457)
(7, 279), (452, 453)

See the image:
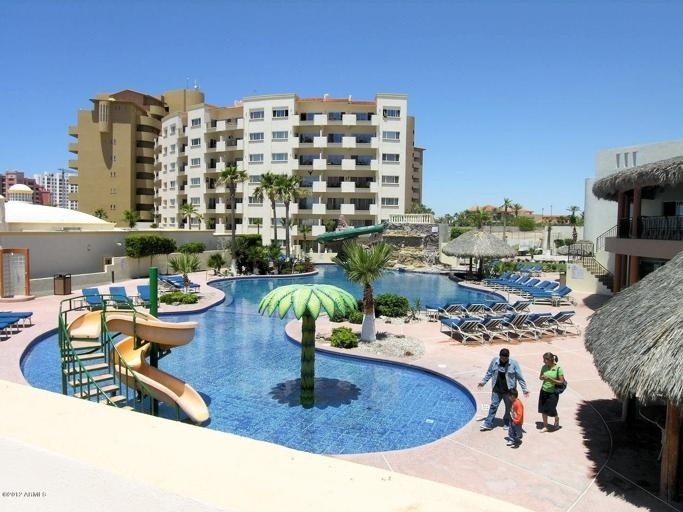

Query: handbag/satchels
(554, 379), (567, 394)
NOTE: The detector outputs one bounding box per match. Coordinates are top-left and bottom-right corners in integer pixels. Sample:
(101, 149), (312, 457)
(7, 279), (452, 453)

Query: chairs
(82, 285), (160, 313)
(482, 263), (572, 305)
(425, 300), (577, 345)
(0, 312), (32, 338)
(157, 275), (200, 293)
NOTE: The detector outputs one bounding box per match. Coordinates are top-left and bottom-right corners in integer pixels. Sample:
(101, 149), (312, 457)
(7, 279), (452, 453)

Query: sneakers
(503, 425), (509, 430)
(480, 423), (492, 430)
(553, 417), (559, 429)
(538, 428), (548, 433)
(506, 441), (519, 448)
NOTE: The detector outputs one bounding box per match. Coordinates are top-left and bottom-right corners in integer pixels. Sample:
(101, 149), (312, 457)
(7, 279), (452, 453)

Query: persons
(538, 352), (564, 432)
(477, 348), (529, 431)
(505, 388), (523, 448)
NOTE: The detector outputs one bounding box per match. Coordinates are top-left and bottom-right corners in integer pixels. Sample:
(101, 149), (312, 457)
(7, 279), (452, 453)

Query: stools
(72, 299), (84, 310)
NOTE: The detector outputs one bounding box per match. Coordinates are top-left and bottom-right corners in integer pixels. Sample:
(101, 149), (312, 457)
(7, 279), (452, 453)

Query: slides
(317, 223), (384, 242)
(67, 309), (209, 425)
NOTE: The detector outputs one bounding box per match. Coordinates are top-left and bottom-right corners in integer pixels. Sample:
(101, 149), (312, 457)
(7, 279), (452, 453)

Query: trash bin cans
(54, 272), (71, 295)
